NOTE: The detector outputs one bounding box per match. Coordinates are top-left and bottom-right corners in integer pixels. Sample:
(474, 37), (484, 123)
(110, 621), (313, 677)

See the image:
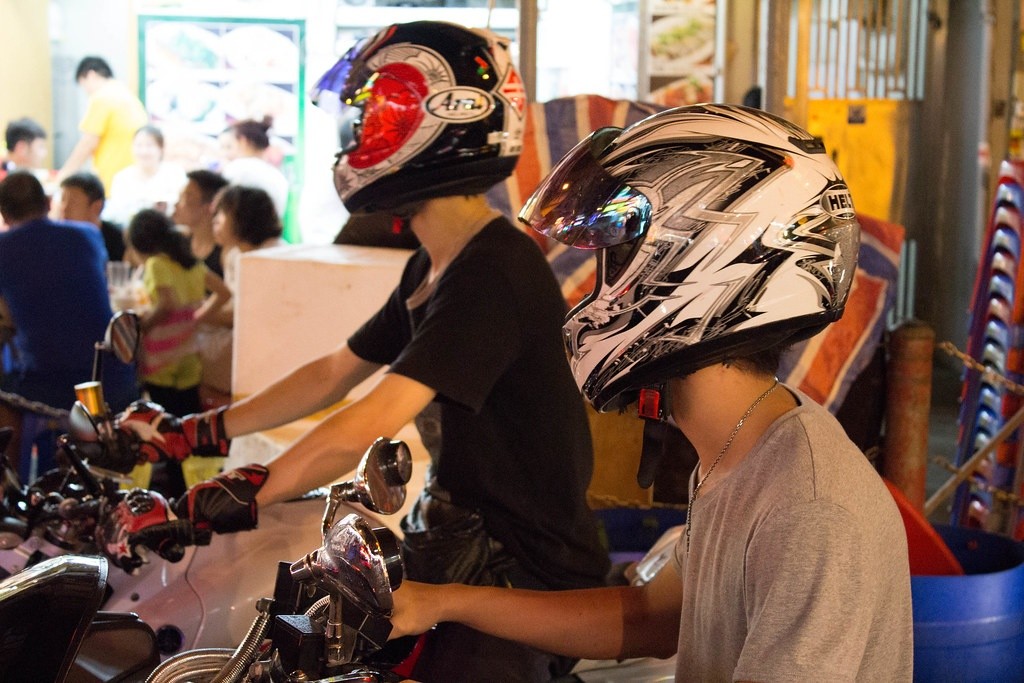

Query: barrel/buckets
(910, 525), (1024, 683)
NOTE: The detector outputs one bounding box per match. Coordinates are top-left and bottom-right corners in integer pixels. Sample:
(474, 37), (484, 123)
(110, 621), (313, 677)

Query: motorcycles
(0, 306), (684, 683)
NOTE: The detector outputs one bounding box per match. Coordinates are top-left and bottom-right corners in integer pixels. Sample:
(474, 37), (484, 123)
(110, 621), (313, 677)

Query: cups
(106, 261), (131, 291)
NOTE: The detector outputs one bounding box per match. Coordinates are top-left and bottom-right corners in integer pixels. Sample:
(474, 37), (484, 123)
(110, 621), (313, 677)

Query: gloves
(114, 399), (232, 463)
(98, 463), (269, 577)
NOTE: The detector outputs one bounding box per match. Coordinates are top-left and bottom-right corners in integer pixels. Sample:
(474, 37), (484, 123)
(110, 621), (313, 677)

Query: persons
(91, 20), (614, 683)
(0, 55), (290, 488)
(385, 101), (915, 683)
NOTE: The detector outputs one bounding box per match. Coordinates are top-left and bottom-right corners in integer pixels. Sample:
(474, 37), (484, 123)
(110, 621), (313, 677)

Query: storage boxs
(224, 243), (432, 541)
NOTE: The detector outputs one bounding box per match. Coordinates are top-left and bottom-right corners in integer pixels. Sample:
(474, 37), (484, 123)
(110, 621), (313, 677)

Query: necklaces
(685, 377), (779, 557)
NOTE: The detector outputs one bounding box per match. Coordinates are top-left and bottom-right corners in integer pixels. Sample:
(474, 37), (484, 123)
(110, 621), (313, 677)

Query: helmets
(560, 103), (861, 412)
(330, 21), (530, 215)
